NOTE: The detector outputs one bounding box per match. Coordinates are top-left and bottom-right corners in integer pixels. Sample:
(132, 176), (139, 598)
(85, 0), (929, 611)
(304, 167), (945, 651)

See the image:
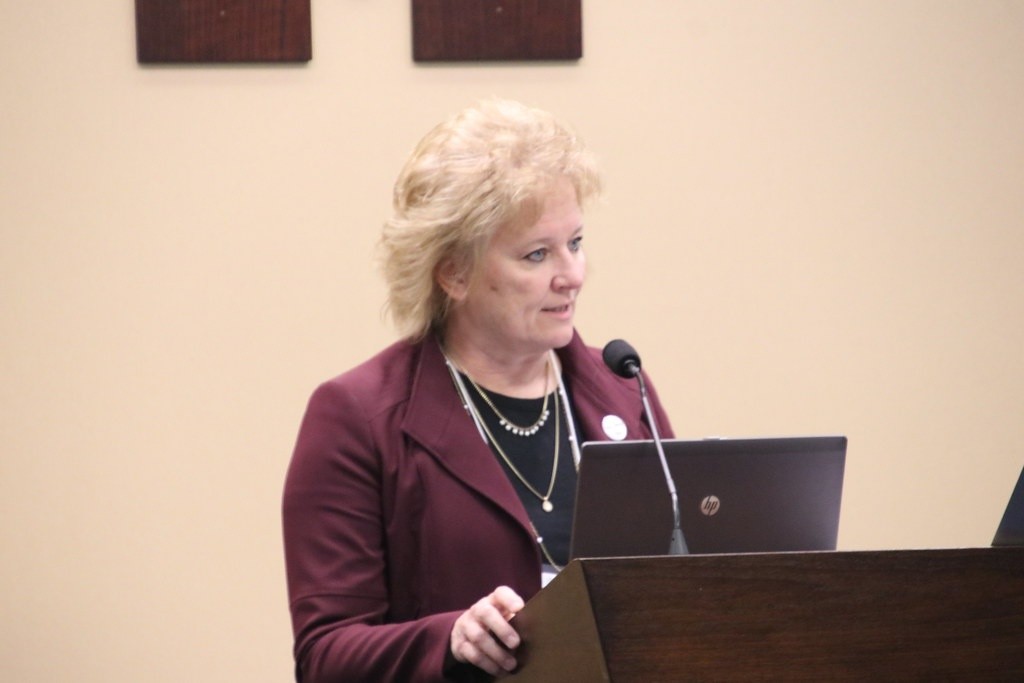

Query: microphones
(600, 339), (690, 558)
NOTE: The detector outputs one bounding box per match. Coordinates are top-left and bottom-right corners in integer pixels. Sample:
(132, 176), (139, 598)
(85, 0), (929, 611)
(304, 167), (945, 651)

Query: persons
(283, 102), (678, 683)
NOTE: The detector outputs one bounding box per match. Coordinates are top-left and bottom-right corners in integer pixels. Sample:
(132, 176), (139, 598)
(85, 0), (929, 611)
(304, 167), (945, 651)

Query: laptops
(569, 436), (847, 562)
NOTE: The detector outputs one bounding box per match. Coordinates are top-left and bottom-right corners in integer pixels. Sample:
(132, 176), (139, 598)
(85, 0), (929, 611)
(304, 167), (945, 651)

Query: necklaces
(443, 354), (586, 573)
